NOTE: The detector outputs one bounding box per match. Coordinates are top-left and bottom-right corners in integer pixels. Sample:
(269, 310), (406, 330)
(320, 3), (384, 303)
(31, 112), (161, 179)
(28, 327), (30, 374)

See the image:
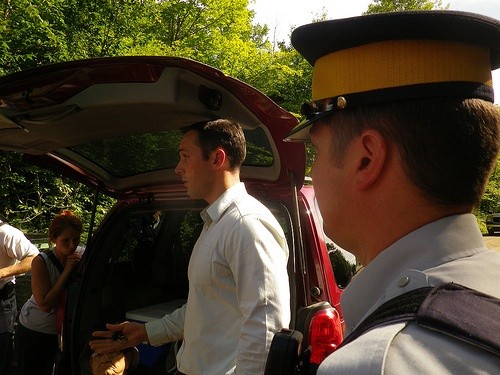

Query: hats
(282, 10), (500, 143)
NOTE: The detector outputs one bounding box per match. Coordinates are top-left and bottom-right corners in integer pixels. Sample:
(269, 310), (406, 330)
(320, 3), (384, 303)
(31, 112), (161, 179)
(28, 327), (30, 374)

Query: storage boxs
(125, 298), (186, 370)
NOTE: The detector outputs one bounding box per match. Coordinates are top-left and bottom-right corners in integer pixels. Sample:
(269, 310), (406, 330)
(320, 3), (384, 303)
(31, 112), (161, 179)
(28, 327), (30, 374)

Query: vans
(0, 56), (361, 375)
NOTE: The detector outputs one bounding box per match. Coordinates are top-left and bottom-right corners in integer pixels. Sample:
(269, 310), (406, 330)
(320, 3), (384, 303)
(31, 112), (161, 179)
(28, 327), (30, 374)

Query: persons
(89, 120), (291, 375)
(0, 219), (40, 375)
(291, 11), (500, 375)
(15, 214), (85, 375)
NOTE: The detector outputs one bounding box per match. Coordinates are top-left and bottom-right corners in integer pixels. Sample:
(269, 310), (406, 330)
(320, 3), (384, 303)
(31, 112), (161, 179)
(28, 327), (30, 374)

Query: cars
(486, 205), (500, 236)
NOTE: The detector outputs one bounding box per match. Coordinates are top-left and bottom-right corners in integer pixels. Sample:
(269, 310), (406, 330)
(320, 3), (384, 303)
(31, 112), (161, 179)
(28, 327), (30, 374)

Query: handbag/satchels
(266, 328), (311, 375)
